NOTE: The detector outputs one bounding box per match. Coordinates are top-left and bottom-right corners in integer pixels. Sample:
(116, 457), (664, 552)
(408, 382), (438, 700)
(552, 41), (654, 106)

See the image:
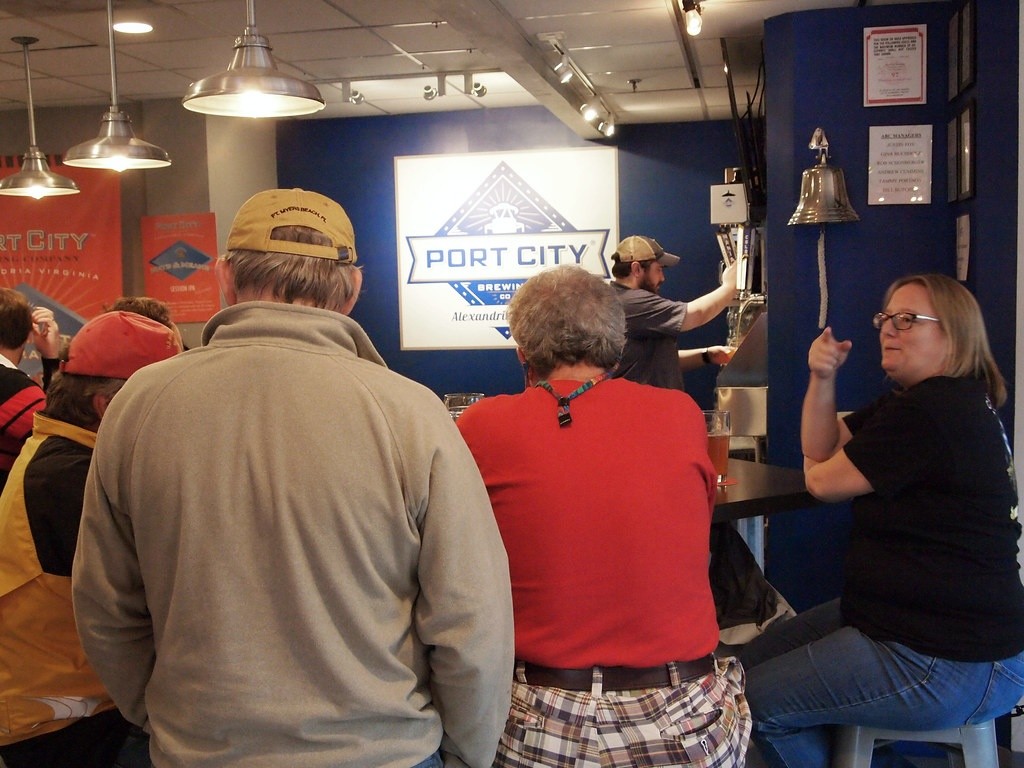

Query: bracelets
(701, 347), (712, 366)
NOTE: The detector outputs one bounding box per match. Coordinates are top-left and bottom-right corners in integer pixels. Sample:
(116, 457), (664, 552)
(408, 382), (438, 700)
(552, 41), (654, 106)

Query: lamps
(342, 81), (364, 105)
(581, 95), (599, 120)
(598, 113), (615, 137)
(463, 73), (487, 97)
(0, 37), (81, 199)
(61, 0), (173, 173)
(423, 75), (447, 100)
(554, 54), (573, 85)
(181, 0), (327, 118)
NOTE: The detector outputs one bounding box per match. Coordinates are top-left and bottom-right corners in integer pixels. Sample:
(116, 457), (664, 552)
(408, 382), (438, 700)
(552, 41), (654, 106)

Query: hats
(613, 234), (681, 267)
(227, 187), (357, 264)
(60, 311), (183, 379)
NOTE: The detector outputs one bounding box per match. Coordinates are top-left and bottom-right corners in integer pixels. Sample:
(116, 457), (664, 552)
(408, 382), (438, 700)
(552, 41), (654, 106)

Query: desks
(710, 459), (824, 524)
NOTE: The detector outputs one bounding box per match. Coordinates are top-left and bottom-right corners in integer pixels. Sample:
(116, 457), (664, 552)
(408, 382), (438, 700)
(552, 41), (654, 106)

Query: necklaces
(534, 362), (620, 428)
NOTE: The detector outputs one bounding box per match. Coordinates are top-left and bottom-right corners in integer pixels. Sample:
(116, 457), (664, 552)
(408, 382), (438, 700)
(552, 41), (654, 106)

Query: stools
(829, 718), (1001, 768)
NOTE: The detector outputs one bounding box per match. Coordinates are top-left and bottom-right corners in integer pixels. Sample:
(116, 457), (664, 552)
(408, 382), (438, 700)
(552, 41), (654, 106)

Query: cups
(702, 410), (731, 483)
(444, 393), (485, 423)
(717, 338), (741, 363)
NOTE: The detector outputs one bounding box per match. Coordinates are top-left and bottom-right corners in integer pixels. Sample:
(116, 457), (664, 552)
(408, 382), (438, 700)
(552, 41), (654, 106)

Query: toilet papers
(729, 388), (767, 436)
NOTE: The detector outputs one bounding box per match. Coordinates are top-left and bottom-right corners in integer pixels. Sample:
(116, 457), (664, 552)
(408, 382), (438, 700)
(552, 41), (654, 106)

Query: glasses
(872, 310), (944, 330)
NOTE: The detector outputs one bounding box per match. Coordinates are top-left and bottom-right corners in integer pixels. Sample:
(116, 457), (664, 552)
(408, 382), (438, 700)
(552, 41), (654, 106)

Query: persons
(450, 266), (751, 768)
(723, 275), (1024, 768)
(606, 235), (737, 391)
(70, 188), (515, 768)
(0, 285), (183, 768)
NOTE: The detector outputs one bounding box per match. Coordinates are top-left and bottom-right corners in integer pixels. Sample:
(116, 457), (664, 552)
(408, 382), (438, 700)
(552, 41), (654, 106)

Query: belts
(511, 655), (715, 693)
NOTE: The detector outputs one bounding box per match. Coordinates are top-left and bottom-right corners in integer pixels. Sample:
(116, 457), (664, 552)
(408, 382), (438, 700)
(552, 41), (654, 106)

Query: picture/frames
(947, 0), (976, 203)
